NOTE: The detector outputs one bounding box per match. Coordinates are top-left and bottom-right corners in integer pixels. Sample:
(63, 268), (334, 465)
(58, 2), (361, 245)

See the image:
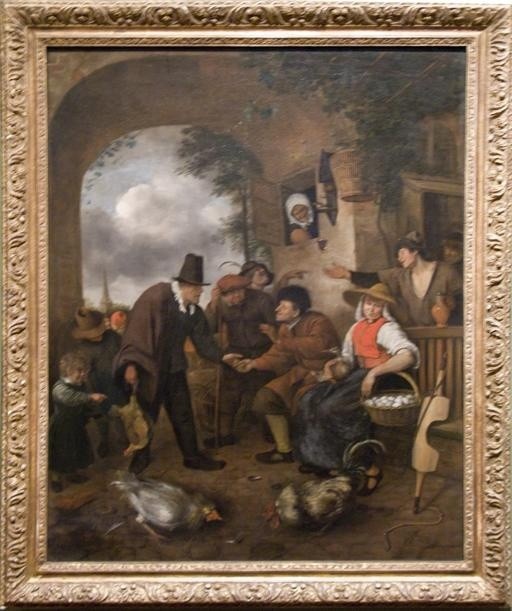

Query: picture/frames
(0, 0), (512, 611)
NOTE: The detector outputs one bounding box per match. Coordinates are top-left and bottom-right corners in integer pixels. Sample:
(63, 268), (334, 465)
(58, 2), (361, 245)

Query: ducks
(309, 345), (352, 382)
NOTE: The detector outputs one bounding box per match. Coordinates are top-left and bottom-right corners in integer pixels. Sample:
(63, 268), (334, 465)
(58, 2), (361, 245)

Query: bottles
(430, 292), (450, 327)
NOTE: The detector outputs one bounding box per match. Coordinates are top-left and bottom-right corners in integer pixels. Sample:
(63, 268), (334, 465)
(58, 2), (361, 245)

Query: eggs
(364, 394), (413, 407)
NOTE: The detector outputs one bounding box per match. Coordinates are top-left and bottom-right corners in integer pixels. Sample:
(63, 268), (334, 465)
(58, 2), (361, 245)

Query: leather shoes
(184, 434), (236, 470)
(256, 431), (320, 473)
(358, 470), (381, 495)
(53, 442), (150, 492)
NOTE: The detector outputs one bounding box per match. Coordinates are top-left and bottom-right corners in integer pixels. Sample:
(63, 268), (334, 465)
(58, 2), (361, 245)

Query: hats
(70, 308), (106, 339)
(173, 253), (211, 285)
(343, 283), (396, 308)
(218, 260), (273, 293)
(398, 231), (426, 248)
(442, 232), (463, 248)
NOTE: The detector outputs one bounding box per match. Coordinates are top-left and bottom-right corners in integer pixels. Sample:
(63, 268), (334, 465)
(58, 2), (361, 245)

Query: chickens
(261, 436), (388, 540)
(105, 380), (150, 462)
(107, 474), (226, 540)
